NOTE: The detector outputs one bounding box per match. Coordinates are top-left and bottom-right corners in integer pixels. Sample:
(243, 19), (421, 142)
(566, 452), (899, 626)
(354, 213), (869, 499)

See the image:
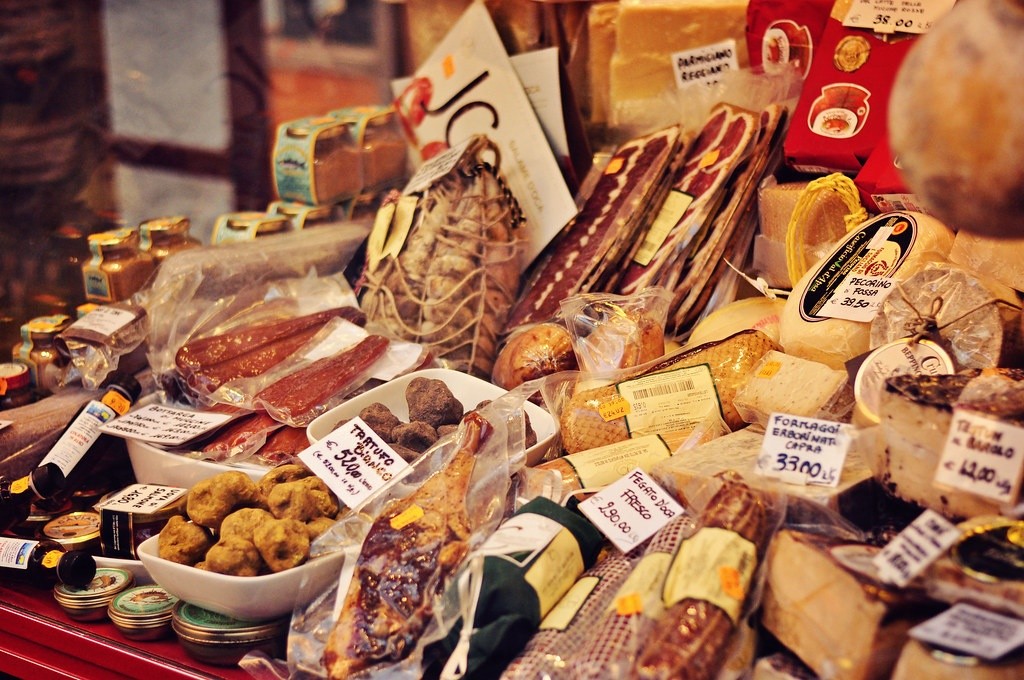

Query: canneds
(0, 107), (407, 412)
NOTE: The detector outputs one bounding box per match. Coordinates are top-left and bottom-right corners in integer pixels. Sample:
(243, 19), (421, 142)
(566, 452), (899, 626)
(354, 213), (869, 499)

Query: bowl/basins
(171, 602), (288, 666)
(107, 584), (179, 640)
(306, 367), (559, 496)
(123, 436), (270, 489)
(137, 519), (365, 623)
(92, 553), (155, 586)
(53, 568), (132, 622)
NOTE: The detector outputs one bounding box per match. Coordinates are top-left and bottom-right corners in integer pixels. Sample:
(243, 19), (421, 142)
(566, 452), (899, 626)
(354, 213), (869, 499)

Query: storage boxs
(758, 528), (940, 680)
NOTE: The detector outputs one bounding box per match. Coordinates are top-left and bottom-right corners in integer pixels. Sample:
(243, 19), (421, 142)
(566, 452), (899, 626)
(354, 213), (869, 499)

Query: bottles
(0, 533), (94, 593)
(37, 372), (139, 515)
(1, 462), (65, 536)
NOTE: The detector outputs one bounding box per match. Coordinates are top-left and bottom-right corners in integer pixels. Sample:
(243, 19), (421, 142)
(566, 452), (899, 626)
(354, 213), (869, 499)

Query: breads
(587, 0), (750, 130)
(491, 178), (1024, 680)
